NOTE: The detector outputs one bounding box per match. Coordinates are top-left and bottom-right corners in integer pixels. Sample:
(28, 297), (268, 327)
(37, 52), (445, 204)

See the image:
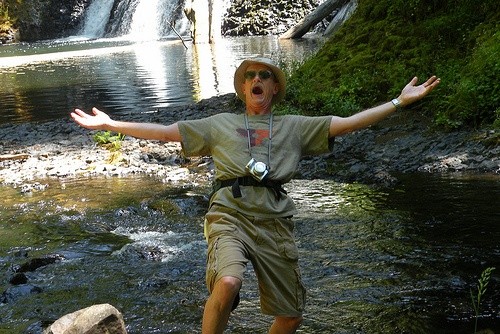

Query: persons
(71, 58), (441, 334)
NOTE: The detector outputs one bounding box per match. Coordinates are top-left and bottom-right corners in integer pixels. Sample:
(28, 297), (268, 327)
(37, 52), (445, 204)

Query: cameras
(245, 158), (268, 182)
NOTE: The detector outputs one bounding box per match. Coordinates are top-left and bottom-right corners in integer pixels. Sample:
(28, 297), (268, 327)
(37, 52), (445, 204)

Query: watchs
(392, 99), (400, 112)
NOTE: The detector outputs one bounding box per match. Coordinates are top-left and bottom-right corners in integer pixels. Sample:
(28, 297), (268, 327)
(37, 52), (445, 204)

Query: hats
(233, 57), (287, 104)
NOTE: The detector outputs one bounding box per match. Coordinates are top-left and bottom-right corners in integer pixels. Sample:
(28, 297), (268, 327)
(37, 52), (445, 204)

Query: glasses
(242, 70), (275, 81)
